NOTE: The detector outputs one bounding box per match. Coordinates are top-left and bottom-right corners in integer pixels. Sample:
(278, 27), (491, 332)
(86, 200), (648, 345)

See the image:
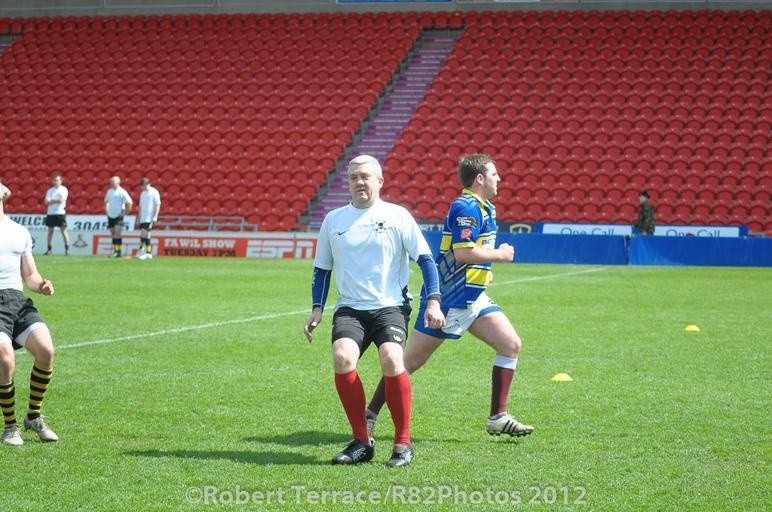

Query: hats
(639, 191), (650, 198)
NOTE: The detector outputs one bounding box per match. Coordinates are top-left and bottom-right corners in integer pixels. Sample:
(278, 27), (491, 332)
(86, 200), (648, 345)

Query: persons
(136, 178), (161, 260)
(632, 191), (656, 236)
(0, 183), (59, 447)
(366, 154), (533, 437)
(303, 155), (446, 468)
(44, 176), (70, 256)
(103, 176), (133, 257)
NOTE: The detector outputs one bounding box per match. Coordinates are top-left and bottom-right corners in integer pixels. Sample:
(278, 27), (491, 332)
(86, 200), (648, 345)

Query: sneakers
(366, 417), (377, 448)
(24, 413), (59, 443)
(332, 438), (375, 466)
(4, 426), (25, 446)
(387, 446), (416, 468)
(486, 415), (535, 438)
